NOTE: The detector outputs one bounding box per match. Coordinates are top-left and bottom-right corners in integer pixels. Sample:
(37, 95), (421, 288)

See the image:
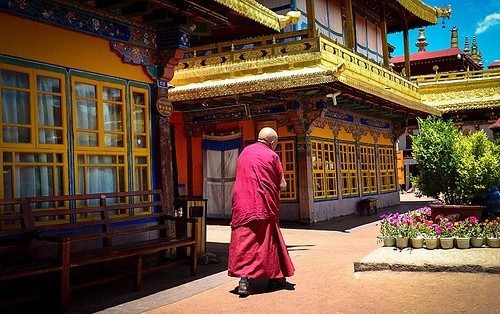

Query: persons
(227, 127), (295, 296)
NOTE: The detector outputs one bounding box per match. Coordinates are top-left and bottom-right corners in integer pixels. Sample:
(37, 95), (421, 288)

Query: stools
(360, 197), (378, 216)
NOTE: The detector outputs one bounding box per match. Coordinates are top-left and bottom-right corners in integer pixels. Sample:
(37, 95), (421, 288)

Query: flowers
(375, 205), (500, 244)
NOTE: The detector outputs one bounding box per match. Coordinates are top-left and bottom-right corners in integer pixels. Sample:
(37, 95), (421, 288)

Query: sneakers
(268, 278), (286, 288)
(238, 282), (255, 294)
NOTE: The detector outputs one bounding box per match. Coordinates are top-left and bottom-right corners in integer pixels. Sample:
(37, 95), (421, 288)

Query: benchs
(0, 190), (199, 314)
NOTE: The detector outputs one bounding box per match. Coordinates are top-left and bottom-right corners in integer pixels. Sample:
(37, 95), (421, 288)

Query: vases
(440, 238), (454, 248)
(472, 237), (483, 247)
(411, 238), (423, 248)
(384, 236), (395, 246)
(425, 238), (438, 249)
(456, 237), (470, 248)
(396, 237), (408, 248)
(488, 237), (500, 247)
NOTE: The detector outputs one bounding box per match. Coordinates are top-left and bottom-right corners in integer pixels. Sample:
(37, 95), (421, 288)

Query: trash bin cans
(175, 198), (207, 256)
